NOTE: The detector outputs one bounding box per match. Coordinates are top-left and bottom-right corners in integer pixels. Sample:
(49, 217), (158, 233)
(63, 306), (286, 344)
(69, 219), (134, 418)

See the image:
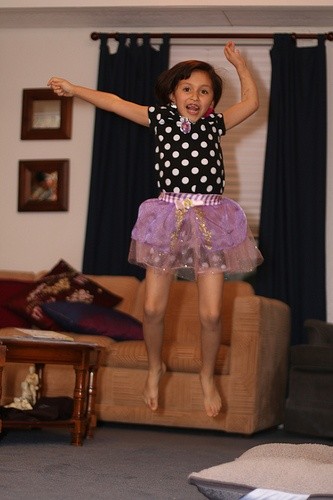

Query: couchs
(0, 270), (289, 435)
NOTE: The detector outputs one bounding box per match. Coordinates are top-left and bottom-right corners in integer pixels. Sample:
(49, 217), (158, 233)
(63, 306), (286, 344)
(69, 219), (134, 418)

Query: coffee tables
(0, 336), (102, 446)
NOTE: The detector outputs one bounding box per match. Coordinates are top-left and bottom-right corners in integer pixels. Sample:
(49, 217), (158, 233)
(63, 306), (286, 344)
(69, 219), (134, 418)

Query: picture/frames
(21, 88), (72, 140)
(18, 160), (70, 212)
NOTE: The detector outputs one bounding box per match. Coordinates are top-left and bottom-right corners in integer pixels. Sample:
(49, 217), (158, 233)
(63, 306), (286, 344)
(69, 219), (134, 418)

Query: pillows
(188, 442), (333, 500)
(0, 258), (143, 339)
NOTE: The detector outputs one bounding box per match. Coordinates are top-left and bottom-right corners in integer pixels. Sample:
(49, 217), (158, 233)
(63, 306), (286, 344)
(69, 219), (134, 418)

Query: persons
(47, 40), (264, 418)
(3, 366), (39, 410)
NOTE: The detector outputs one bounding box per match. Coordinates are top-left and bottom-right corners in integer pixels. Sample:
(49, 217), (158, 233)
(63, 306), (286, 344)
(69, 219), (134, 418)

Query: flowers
(176, 115), (191, 134)
